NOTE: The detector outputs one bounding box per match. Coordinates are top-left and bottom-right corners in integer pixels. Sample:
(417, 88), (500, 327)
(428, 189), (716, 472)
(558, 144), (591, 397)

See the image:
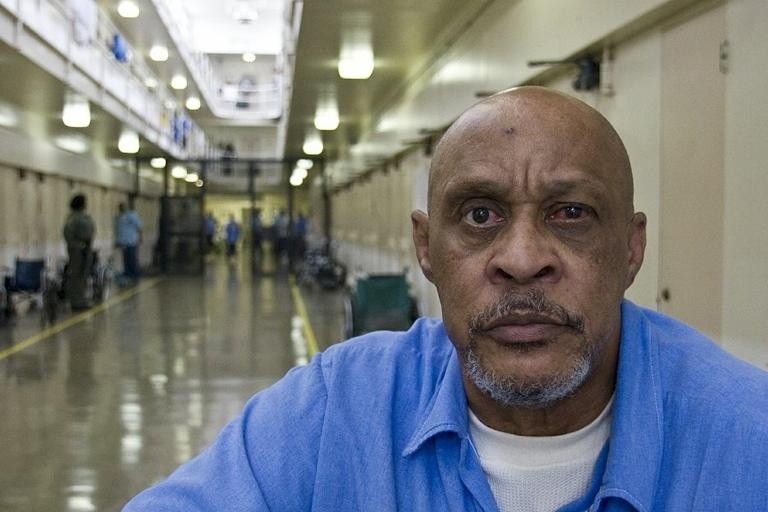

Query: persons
(205, 212), (218, 249)
(62, 193), (97, 310)
(225, 213), (240, 255)
(252, 208), (306, 263)
(120, 85), (768, 512)
(114, 203), (144, 279)
